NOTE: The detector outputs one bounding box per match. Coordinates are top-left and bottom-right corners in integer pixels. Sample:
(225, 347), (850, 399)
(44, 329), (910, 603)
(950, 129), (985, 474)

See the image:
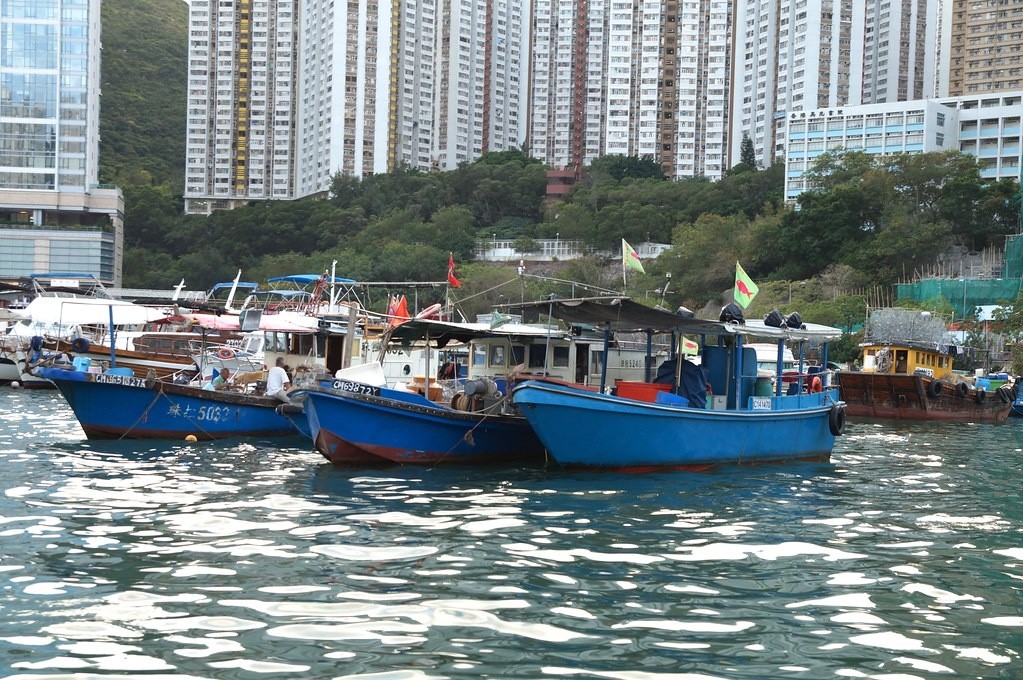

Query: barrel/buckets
(975, 376), (991, 391)
(755, 377), (774, 397)
(616, 381), (672, 404)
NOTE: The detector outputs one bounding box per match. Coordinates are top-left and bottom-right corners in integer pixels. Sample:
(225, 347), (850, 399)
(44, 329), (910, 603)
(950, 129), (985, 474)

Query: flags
(681, 336), (698, 356)
(448, 257), (461, 288)
(734, 261), (759, 309)
(623, 238), (646, 274)
(388, 295), (411, 327)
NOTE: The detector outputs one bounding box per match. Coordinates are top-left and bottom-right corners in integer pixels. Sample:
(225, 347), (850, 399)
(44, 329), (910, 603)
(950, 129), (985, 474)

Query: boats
(0, 259), (1023, 470)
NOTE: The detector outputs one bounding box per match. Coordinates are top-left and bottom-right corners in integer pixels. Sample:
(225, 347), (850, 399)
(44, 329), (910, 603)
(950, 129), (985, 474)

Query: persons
(264, 357), (291, 404)
(212, 368), (231, 391)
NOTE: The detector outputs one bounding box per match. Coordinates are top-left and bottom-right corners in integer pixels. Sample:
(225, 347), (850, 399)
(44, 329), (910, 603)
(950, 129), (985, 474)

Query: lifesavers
(72, 337), (89, 353)
(808, 376), (823, 394)
(996, 388), (1017, 404)
(926, 379), (944, 398)
(829, 405), (845, 436)
(955, 382), (970, 398)
(975, 390), (987, 405)
(414, 303), (442, 319)
(217, 349), (234, 359)
